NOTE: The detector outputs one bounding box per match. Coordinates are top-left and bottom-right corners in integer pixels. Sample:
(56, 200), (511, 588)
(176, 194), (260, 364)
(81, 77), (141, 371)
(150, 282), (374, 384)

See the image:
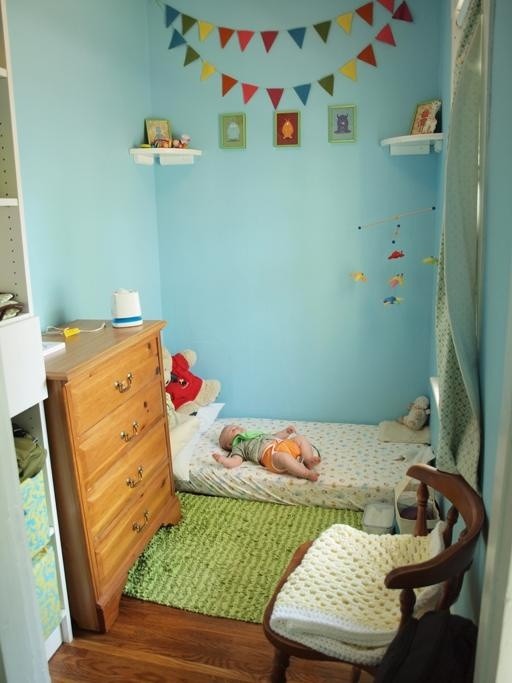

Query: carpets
(123, 490), (363, 625)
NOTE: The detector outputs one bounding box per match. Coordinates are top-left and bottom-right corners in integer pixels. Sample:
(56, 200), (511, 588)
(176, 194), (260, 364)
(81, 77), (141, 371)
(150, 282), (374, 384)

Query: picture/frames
(274, 110), (301, 147)
(220, 112), (247, 149)
(145, 119), (172, 145)
(328, 104), (357, 143)
(408, 98), (441, 134)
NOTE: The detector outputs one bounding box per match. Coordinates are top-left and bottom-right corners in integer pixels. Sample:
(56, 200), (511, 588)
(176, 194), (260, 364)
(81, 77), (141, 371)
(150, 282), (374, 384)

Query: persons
(212, 425), (322, 481)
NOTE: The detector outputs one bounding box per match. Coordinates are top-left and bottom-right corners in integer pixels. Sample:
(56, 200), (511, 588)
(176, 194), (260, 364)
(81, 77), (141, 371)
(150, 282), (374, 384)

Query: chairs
(261, 463), (486, 683)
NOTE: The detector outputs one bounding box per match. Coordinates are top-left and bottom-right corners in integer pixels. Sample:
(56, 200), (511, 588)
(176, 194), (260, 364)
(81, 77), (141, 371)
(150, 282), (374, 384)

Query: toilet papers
(112, 288), (143, 328)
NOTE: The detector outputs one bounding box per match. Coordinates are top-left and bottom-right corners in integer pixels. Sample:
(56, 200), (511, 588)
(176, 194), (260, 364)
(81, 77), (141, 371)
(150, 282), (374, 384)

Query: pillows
(170, 415), (199, 459)
(173, 402), (226, 482)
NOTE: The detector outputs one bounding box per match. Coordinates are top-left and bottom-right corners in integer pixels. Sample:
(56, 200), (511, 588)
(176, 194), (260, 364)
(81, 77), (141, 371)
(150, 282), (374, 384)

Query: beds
(175, 417), (436, 513)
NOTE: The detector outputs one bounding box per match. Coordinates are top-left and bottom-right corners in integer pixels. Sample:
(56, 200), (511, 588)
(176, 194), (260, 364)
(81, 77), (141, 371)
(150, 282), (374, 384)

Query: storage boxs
(395, 447), (441, 534)
(360, 502), (394, 533)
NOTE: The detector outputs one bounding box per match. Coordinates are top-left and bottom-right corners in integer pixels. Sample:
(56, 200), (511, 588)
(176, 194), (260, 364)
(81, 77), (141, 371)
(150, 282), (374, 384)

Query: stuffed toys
(396, 396), (431, 430)
(163, 339), (221, 416)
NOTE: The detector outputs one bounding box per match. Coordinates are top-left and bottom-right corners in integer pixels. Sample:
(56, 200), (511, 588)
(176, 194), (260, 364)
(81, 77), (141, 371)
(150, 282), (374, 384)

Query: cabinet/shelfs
(42, 320), (182, 633)
(2, 3), (75, 683)
